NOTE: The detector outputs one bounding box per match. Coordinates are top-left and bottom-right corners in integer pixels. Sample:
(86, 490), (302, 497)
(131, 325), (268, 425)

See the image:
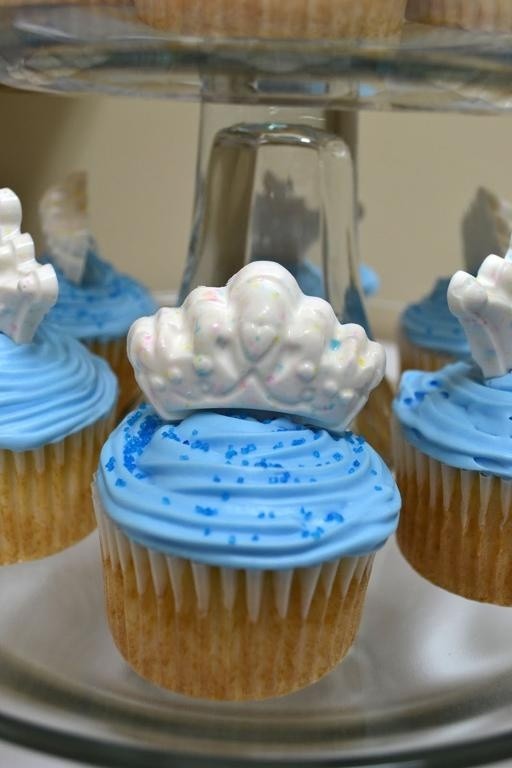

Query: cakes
(90, 261), (402, 700)
(398, 256), (480, 370)
(391, 246), (512, 607)
(34, 185), (158, 411)
(0, 189), (120, 568)
